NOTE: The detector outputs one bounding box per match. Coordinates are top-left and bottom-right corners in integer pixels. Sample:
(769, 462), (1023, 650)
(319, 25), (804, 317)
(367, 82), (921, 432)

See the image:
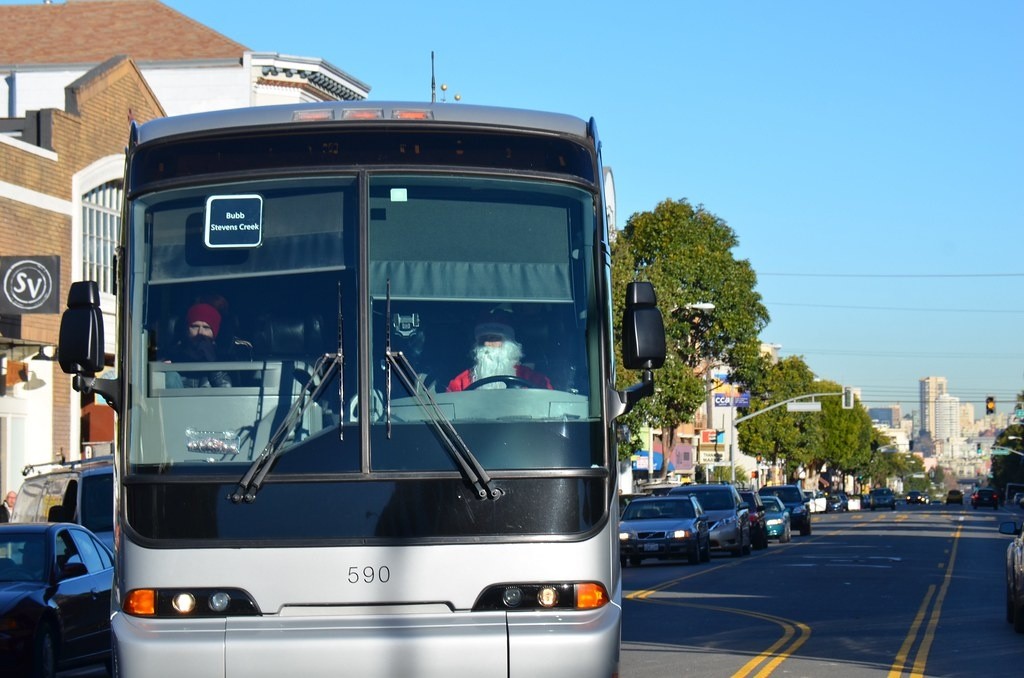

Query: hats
(472, 307), (516, 341)
(184, 303), (222, 339)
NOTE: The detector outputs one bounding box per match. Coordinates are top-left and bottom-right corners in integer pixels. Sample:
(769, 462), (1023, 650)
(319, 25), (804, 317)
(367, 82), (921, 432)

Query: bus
(59, 98), (668, 678)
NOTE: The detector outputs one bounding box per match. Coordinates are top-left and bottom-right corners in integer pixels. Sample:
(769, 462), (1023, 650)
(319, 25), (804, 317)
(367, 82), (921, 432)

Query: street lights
(730, 343), (783, 483)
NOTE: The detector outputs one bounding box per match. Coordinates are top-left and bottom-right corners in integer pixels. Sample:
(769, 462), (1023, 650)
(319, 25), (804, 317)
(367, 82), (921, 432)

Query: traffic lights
(976, 443), (981, 453)
(985, 397), (994, 416)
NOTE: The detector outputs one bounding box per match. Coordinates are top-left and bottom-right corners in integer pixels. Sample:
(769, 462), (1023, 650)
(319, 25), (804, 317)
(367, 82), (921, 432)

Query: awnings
(632, 450), (675, 472)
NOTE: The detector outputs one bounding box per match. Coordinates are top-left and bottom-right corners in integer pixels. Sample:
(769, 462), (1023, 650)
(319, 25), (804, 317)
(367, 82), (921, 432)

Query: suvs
(757, 483), (811, 537)
(7, 453), (116, 567)
(735, 488), (767, 553)
(868, 488), (897, 512)
(944, 488), (964, 505)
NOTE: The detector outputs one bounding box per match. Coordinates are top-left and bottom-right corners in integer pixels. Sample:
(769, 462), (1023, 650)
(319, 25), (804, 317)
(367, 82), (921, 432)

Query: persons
(0, 491), (17, 523)
(154, 299), (231, 388)
(445, 310), (554, 392)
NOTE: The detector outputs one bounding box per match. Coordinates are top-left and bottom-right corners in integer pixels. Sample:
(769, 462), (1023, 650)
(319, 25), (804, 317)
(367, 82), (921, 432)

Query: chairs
(637, 508), (659, 517)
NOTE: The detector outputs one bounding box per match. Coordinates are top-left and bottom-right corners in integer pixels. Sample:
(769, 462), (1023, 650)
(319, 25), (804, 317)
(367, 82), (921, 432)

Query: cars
(970, 487), (1000, 510)
(999, 516), (1024, 634)
(802, 489), (870, 515)
(758, 494), (791, 543)
(618, 492), (711, 569)
(905, 490), (930, 506)
(657, 480), (751, 559)
(0, 522), (117, 678)
